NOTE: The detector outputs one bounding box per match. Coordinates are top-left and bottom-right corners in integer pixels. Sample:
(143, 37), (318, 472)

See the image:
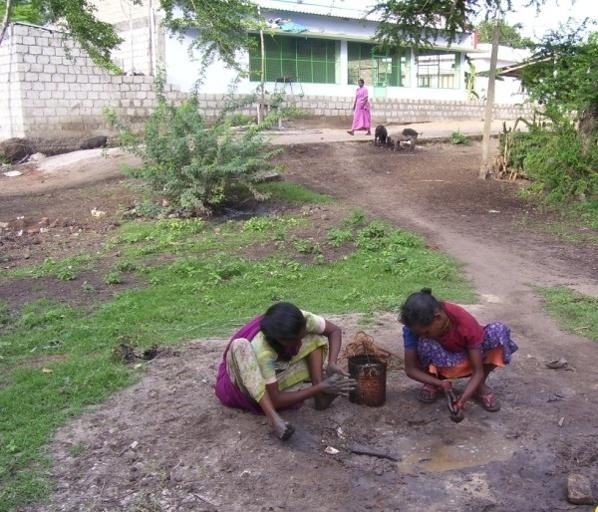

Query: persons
(396, 286), (520, 423)
(214, 300), (360, 442)
(346, 79), (372, 137)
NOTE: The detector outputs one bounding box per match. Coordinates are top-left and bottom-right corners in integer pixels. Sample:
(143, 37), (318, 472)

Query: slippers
(416, 386), (439, 404)
(480, 391), (500, 411)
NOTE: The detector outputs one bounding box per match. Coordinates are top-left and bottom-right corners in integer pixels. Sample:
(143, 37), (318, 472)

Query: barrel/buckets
(348, 345), (388, 407)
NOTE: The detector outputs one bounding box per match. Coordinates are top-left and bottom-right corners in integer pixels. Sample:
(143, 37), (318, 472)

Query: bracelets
(453, 400), (464, 408)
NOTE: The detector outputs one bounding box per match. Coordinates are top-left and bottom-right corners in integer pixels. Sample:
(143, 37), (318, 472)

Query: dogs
(388, 128), (424, 152)
(374, 125), (388, 145)
